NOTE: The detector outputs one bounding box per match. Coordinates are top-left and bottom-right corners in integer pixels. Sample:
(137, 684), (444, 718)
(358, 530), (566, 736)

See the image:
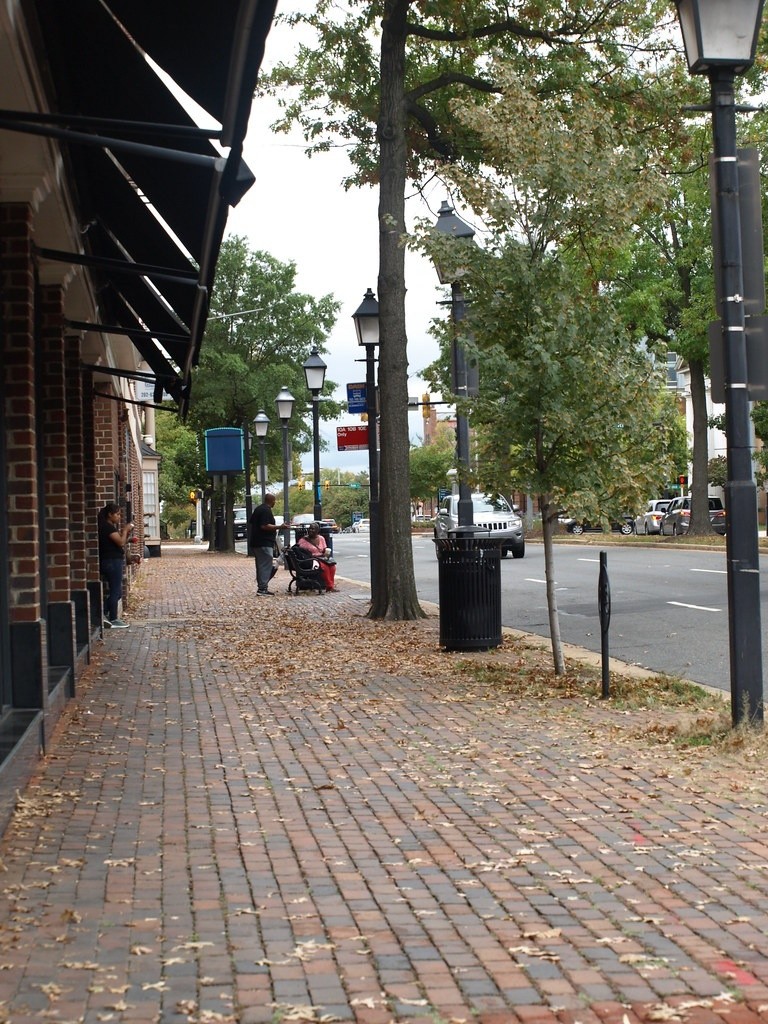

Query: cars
(344, 519), (369, 533)
(291, 513), (314, 534)
(231, 508), (249, 540)
(412, 514), (432, 524)
(558, 515), (572, 524)
(567, 514), (635, 535)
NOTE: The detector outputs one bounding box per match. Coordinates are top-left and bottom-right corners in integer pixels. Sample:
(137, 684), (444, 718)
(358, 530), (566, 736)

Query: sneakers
(103, 615), (112, 627)
(110, 619), (130, 629)
(256, 588), (275, 597)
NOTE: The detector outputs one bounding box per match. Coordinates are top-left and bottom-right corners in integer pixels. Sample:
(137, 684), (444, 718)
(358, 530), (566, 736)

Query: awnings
(1, 0), (277, 423)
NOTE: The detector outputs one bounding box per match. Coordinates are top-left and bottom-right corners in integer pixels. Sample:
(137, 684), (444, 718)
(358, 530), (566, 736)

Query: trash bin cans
(293, 521), (334, 557)
(431, 524), (516, 654)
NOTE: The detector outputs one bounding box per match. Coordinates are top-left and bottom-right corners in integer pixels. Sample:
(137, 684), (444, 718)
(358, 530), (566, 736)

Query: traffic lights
(674, 475), (688, 484)
(325, 481), (329, 491)
(189, 491), (201, 499)
(298, 482), (302, 490)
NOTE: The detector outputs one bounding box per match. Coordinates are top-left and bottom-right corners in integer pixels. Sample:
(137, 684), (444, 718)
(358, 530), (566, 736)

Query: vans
(660, 496), (726, 537)
(273, 516), (285, 535)
(633, 499), (672, 536)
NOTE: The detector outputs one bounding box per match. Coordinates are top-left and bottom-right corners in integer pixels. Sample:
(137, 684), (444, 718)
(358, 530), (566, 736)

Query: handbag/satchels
(315, 556), (337, 565)
(273, 540), (280, 558)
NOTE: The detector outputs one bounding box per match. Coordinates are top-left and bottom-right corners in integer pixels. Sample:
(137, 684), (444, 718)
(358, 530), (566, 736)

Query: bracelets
(127, 529), (130, 534)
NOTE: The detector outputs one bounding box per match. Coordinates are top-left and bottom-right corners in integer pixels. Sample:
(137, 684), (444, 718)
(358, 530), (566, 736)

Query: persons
(251, 494), (290, 596)
(98, 504), (134, 628)
(281, 522), (341, 592)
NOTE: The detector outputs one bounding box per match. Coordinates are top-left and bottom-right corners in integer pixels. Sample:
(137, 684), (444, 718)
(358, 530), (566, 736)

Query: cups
(325, 548), (331, 557)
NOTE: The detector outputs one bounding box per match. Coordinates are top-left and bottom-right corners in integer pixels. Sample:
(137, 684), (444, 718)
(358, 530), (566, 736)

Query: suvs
(323, 518), (337, 533)
(434, 491), (525, 559)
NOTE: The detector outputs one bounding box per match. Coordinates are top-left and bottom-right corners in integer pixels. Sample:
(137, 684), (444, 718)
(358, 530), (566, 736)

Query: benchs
(280, 546), (328, 596)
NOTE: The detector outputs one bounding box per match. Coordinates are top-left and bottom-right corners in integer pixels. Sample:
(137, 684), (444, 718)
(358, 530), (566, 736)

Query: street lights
(274, 386), (296, 551)
(674, 0), (764, 739)
(252, 409), (271, 505)
(242, 432), (252, 521)
(302, 345), (328, 529)
(423, 200), (477, 554)
(351, 288), (391, 620)
